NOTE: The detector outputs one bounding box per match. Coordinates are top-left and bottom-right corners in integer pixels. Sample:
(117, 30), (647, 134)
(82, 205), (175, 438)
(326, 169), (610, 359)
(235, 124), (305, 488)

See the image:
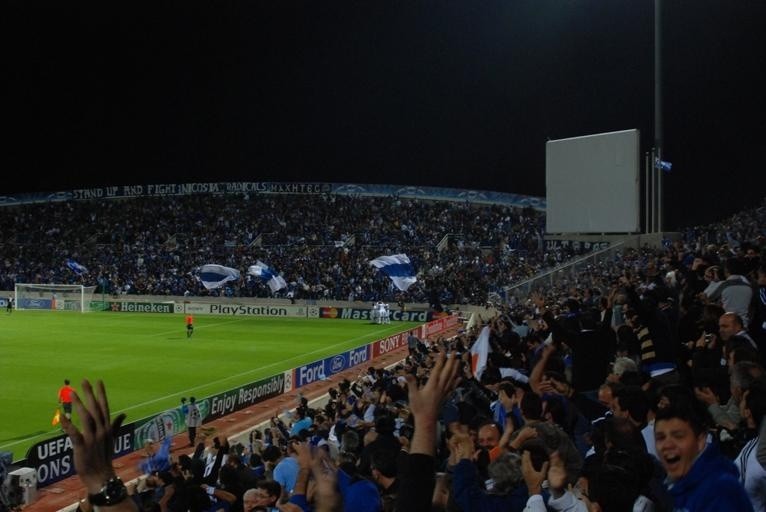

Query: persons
(0, 191), (604, 309)
(186, 313), (194, 337)
(464, 202), (766, 342)
(0, 331), (766, 512)
(6, 294), (15, 315)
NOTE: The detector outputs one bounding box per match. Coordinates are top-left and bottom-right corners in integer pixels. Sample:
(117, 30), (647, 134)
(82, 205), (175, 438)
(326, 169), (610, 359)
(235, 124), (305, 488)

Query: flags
(653, 153), (672, 176)
(52, 410), (61, 426)
